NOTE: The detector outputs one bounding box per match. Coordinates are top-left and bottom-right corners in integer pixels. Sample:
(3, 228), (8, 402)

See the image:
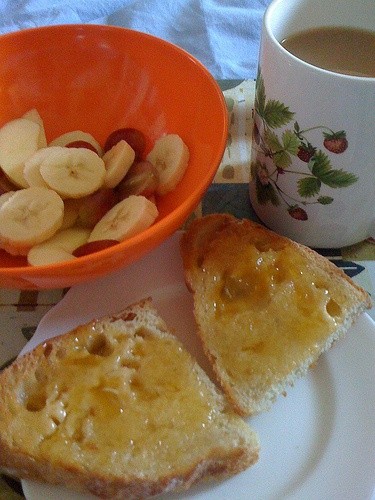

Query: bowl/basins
(0, 23), (227, 284)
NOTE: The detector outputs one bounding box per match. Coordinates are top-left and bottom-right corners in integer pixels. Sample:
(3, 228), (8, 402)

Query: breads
(0, 295), (261, 500)
(180, 213), (372, 419)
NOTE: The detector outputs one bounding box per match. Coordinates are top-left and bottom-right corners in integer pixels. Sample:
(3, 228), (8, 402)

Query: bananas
(0, 107), (189, 268)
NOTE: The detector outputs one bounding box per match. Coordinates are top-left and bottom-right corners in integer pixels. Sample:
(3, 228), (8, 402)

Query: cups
(250, 0), (374, 252)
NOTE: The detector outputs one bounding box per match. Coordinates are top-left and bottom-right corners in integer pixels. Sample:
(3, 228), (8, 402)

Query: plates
(15, 227), (375, 499)
